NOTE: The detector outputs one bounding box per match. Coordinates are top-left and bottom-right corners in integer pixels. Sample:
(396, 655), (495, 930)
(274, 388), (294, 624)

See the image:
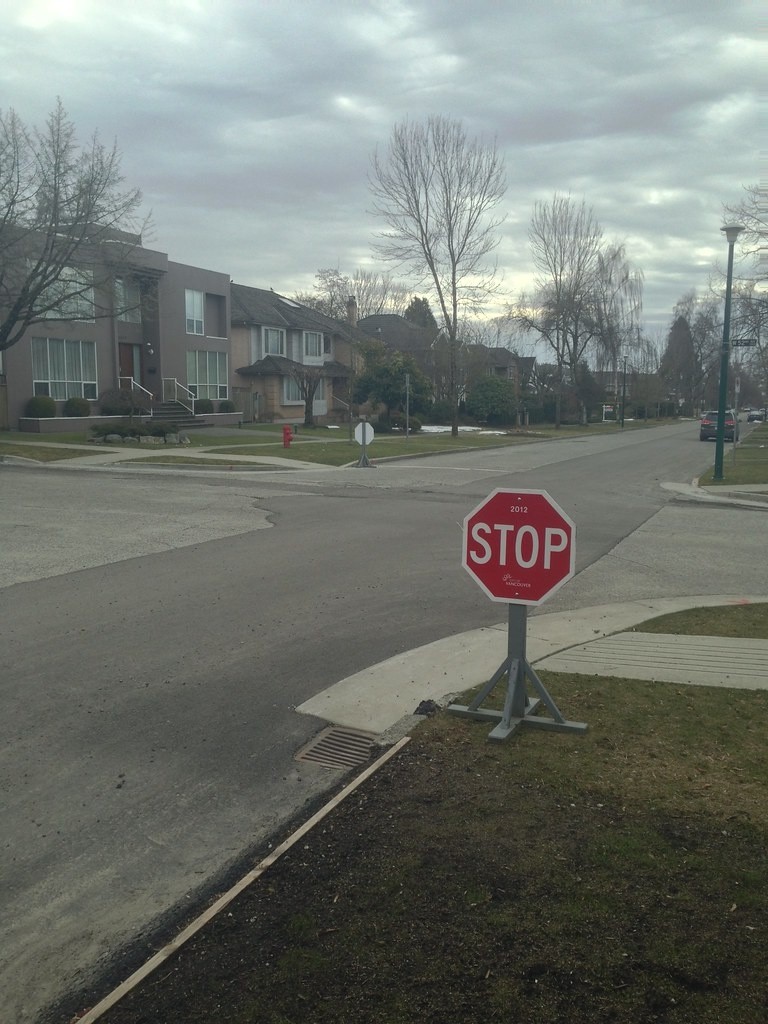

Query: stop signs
(461, 489), (578, 607)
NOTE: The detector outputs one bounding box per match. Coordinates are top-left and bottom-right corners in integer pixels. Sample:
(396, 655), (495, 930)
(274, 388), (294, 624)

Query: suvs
(700, 411), (744, 443)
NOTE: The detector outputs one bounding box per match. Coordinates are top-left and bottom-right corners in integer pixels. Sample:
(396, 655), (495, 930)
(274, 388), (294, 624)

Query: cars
(744, 407), (750, 412)
(748, 411), (764, 422)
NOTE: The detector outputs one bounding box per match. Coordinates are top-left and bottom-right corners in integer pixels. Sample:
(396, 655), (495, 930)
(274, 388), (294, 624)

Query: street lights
(714, 223), (747, 482)
(621, 355), (629, 428)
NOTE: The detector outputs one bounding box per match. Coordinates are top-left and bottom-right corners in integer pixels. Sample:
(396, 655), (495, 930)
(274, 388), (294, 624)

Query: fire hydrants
(282, 423), (295, 449)
